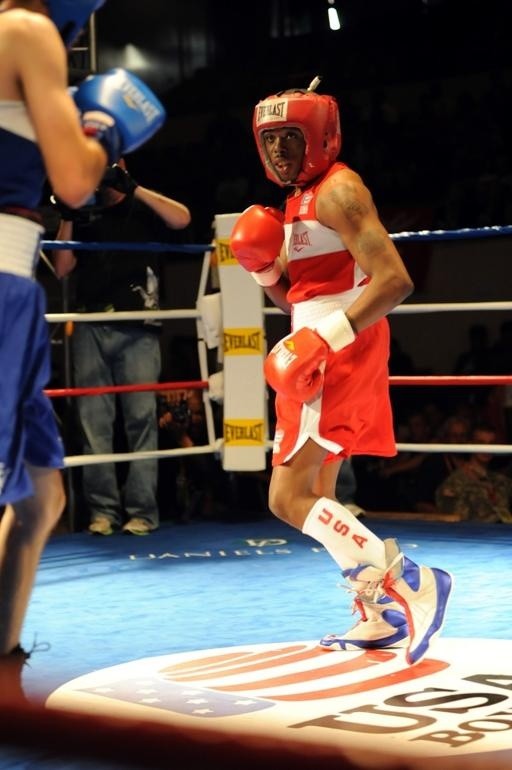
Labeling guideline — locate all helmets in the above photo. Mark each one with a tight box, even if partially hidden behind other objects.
[40,0,105,48]
[249,87,342,188]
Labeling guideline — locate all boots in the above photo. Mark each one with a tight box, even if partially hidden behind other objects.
[347,535,455,668]
[317,563,410,656]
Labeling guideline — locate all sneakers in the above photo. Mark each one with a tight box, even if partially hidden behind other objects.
[122,515,155,538]
[87,516,114,537]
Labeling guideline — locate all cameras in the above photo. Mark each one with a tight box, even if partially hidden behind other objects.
[160,394,193,429]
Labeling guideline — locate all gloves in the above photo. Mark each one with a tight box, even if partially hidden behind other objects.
[101,163,138,196]
[70,67,167,169]
[262,309,357,406]
[228,204,287,287]
[50,193,81,221]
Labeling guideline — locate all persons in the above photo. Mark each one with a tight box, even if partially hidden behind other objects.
[229,86,452,664]
[50,156,191,536]
[0,0,169,665]
